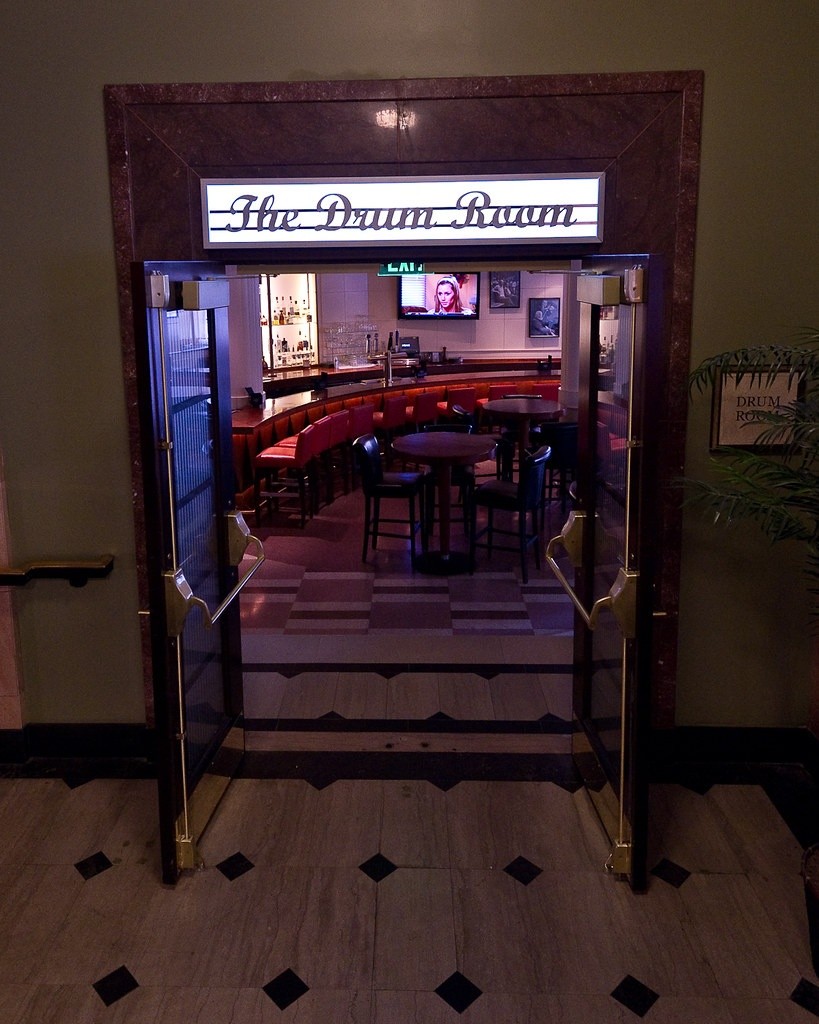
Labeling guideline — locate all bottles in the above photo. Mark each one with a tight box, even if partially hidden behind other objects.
[600,334,615,364]
[273,296,315,367]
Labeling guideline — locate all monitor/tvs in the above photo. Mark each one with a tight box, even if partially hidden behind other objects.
[399,336,420,354]
[397,271,482,320]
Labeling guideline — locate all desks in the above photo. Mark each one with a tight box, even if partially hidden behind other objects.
[230,369,560,507]
[482,398,563,482]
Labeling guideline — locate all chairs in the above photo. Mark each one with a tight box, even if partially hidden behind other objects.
[524,421,579,513]
[422,424,474,535]
[253,382,551,529]
[468,445,551,584]
[352,433,426,577]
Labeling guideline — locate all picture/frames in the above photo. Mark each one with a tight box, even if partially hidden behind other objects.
[489,271,521,309]
[528,297,561,338]
[709,364,807,456]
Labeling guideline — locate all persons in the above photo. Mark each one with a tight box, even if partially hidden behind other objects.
[492,272,519,305]
[427,276,471,314]
[532,300,556,335]
